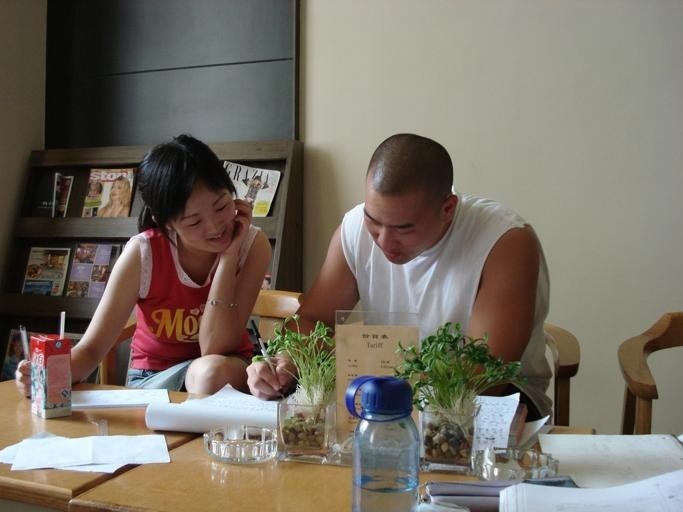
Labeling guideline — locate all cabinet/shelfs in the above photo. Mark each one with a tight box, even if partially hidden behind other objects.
[0,138,303,381]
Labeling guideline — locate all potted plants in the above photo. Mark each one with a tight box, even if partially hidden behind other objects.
[252,316,336,463]
[392,323,528,464]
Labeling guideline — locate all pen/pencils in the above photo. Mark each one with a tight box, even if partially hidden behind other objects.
[20,324,30,360]
[251,319,284,400]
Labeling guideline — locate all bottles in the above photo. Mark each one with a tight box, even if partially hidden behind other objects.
[344,375,421,511]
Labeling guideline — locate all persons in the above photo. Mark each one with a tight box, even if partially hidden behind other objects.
[244,131,558,425]
[12,133,272,401]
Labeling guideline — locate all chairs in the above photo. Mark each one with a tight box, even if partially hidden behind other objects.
[616,311,683,434]
[98,288,301,386]
[542,322,580,429]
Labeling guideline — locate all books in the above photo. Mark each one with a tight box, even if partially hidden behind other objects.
[64,241,127,299]
[220,157,281,218]
[67,387,172,412]
[497,473,682,512]
[20,246,71,298]
[79,167,138,218]
[412,385,532,457]
[420,476,572,510]
[143,379,286,438]
[203,297,239,313]
[0,325,41,383]
[58,329,104,385]
[28,172,73,219]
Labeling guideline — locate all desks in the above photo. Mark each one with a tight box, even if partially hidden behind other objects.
[68,428,615,512]
[0,376,210,512]
